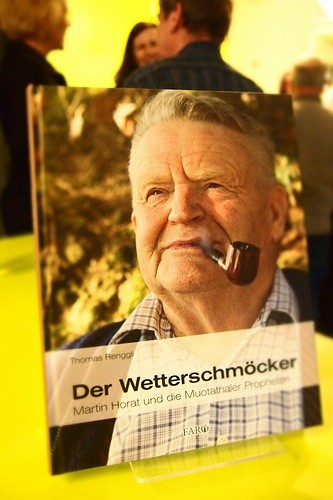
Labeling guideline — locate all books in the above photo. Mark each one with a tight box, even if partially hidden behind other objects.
[25,83,325,474]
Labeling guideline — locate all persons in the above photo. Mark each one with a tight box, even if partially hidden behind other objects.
[51,91,322,476]
[114,22,163,89]
[280,52,333,339]
[120,0,264,93]
[0,0,70,238]
